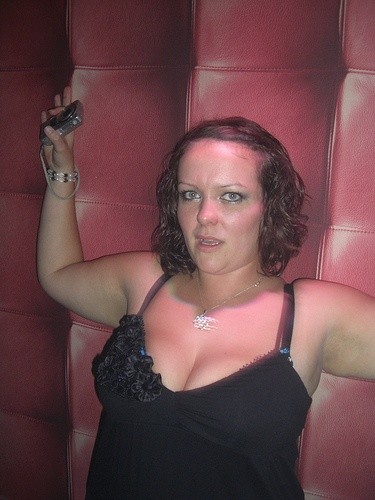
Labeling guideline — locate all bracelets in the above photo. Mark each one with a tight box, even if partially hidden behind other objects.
[45,170,77,185]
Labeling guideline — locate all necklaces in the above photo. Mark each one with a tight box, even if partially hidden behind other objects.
[193,269,261,331]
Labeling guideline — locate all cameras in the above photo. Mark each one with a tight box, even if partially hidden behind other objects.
[39,100,83,146]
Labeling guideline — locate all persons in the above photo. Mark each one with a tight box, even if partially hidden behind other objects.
[36,86,375,499]
